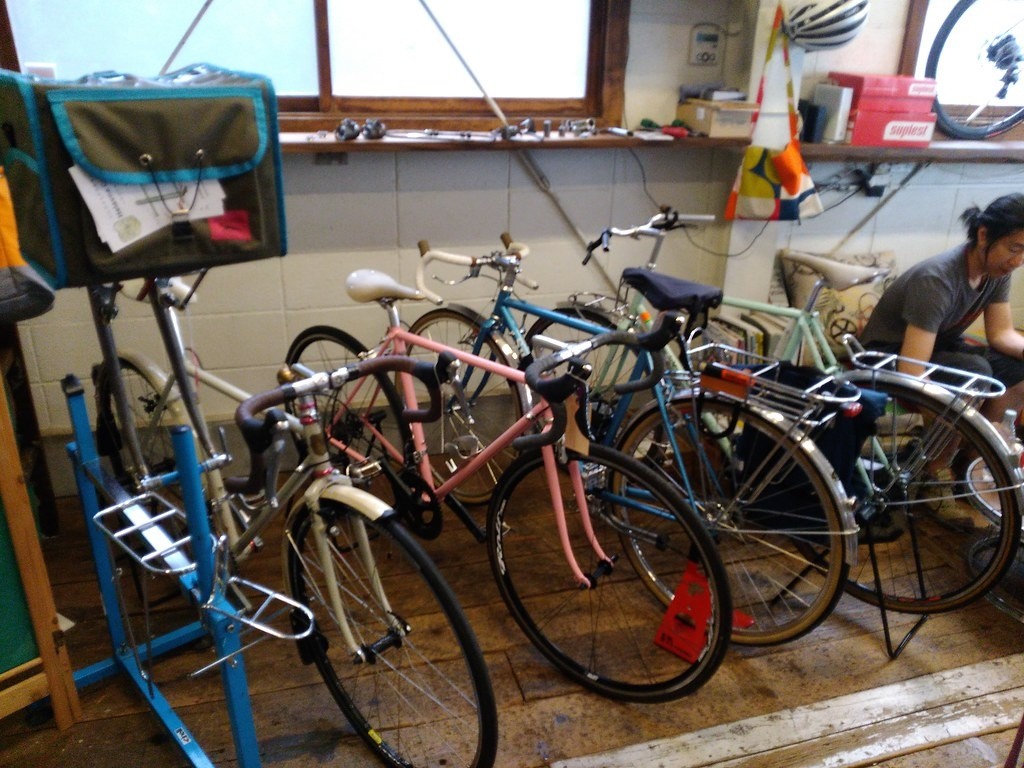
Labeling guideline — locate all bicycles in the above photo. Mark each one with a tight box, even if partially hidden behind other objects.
[91,274,500,768]
[524,203,1024,658]
[404,231,862,647]
[278,268,734,703]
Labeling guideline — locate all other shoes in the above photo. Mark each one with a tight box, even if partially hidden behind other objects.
[916,468,974,525]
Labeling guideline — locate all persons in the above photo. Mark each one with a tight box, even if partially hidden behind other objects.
[859,192,1024,515]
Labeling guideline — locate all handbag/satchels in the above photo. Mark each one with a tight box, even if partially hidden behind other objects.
[724,142,825,219]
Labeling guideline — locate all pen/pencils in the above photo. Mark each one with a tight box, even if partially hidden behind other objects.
[608,126,633,137]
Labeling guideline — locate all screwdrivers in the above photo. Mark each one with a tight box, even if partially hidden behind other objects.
[636,125,691,138]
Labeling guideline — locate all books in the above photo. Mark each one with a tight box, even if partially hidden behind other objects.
[702,309,805,366]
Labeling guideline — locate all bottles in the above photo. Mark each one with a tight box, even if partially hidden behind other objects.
[983,410,1017,482]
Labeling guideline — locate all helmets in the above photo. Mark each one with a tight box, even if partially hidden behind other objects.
[780,0,870,52]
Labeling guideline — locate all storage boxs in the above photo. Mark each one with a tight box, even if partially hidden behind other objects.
[842,109,938,148]
[676,99,761,138]
[829,72,939,113]
